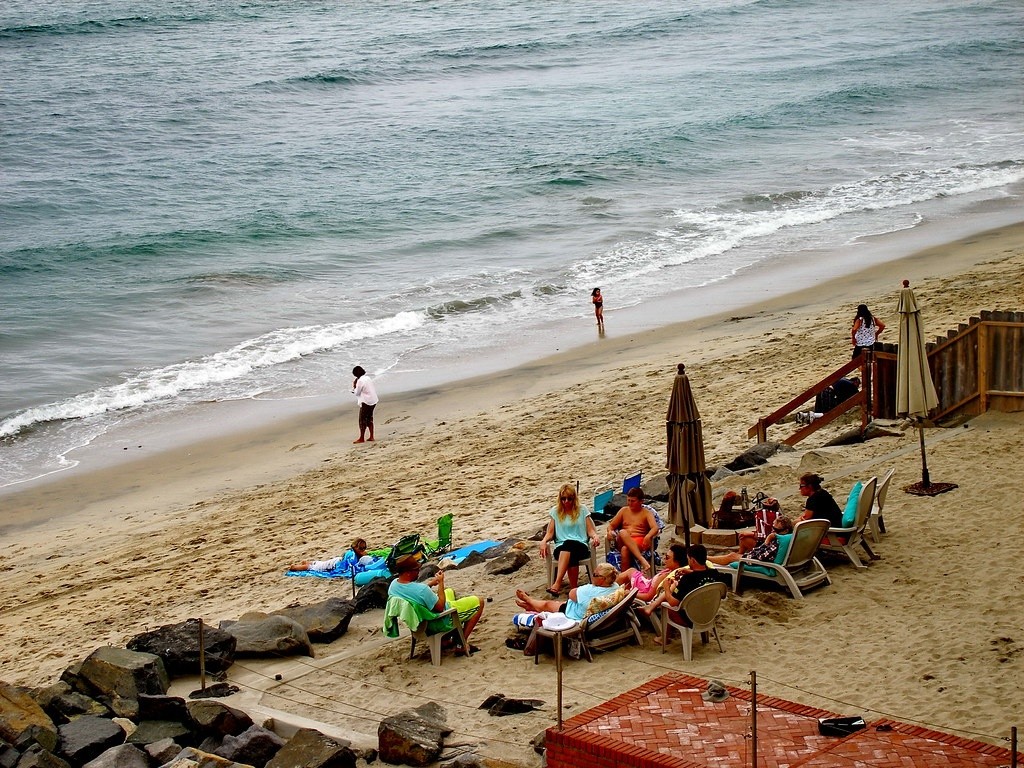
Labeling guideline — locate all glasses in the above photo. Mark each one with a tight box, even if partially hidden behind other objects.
[666,555,676,560]
[561,497,573,501]
[359,546,366,550]
[877,724,893,731]
[800,484,808,488]
[772,526,787,535]
[593,573,606,580]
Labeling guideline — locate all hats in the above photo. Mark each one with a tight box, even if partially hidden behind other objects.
[396,554,422,570]
[701,679,729,702]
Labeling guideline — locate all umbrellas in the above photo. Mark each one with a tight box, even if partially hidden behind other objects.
[895,279,939,486]
[664,361,715,544]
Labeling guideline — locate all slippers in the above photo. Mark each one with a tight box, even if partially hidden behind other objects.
[545,588,559,598]
[442,641,455,650]
[455,644,481,657]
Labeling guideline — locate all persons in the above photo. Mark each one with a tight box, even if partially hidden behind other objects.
[616,542,688,600]
[513,563,621,620]
[591,288,604,325]
[706,514,794,567]
[352,366,379,443]
[636,544,727,644]
[790,472,848,545]
[795,375,862,423]
[387,554,484,656]
[288,537,367,571]
[538,483,600,598]
[605,487,660,570]
[849,303,886,371]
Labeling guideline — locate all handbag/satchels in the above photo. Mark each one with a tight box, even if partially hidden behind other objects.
[711,510,756,529]
[751,492,785,516]
[818,716,867,735]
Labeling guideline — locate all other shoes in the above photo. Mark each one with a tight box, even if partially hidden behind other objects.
[505,638,527,650]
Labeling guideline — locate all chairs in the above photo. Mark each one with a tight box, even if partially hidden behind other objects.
[353,468,896,666]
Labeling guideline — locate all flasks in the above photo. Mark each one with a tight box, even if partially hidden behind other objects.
[741,486,749,510]
